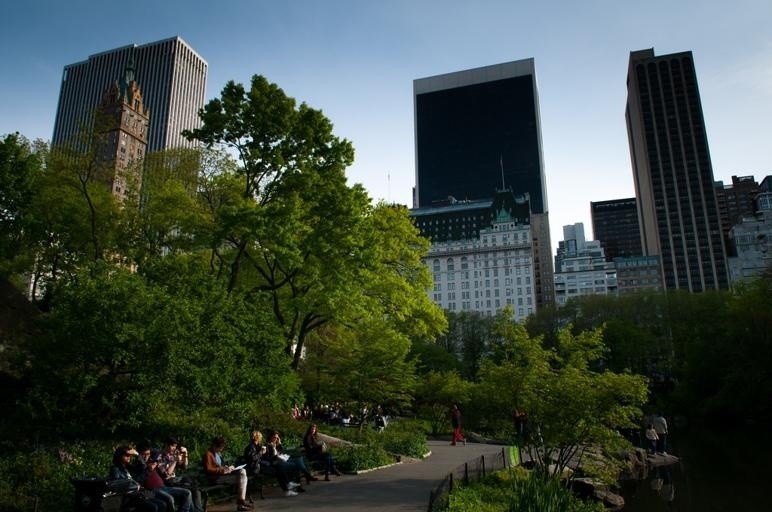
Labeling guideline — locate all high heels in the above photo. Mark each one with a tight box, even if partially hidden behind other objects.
[305,477,318,485]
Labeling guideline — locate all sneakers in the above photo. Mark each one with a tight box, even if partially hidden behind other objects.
[297,487,307,493]
[237,499,253,511]
[287,482,301,489]
[324,471,342,481]
[282,490,299,496]
[451,438,466,445]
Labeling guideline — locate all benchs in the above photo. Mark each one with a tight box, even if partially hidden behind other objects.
[195,476,266,512]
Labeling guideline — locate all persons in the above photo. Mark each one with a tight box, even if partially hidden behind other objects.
[645,422,659,455]
[292,400,390,433]
[515,406,529,440]
[450,404,466,445]
[109,422,344,512]
[654,408,668,456]
